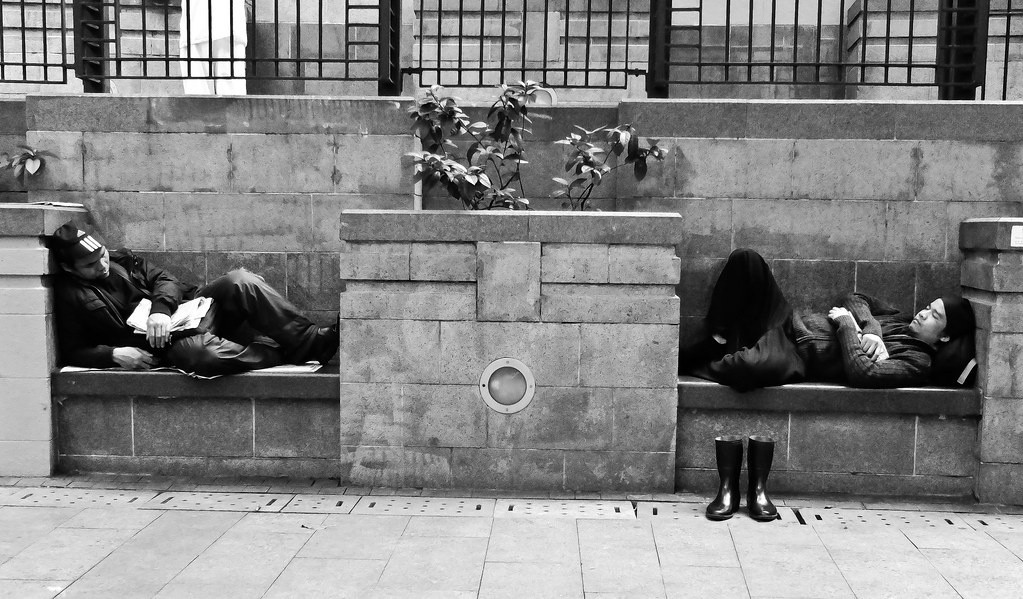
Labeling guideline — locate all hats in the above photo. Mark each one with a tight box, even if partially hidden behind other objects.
[50,218,106,263]
[938,295,975,342]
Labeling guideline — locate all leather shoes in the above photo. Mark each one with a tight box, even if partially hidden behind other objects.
[309,311,339,367]
[254,335,303,364]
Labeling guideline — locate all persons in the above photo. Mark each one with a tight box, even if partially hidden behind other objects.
[678,247,976,393]
[52,219,341,376]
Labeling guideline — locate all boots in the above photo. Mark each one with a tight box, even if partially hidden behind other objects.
[707,434,743,520]
[746,435,778,521]
